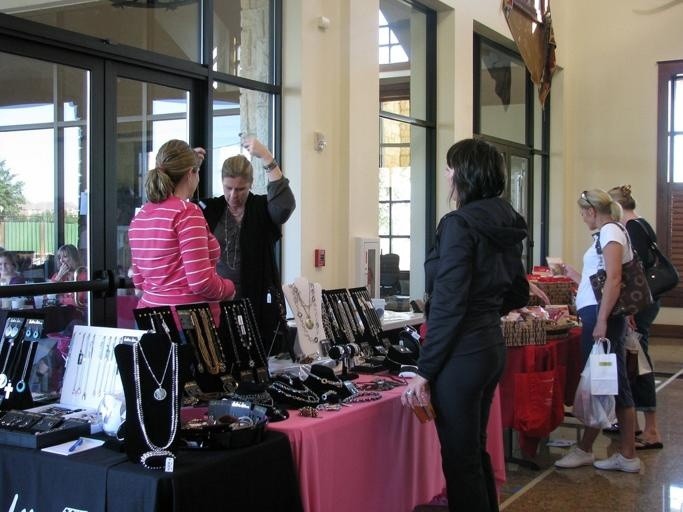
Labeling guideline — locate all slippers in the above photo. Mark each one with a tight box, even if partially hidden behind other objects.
[602,423,642,436]
[634,437,663,450]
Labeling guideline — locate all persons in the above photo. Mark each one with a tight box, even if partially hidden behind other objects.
[127,140,237,332]
[47,244,88,306]
[554,188,642,474]
[198,131,295,335]
[607,184,664,452]
[0,250,37,308]
[401,140,533,512]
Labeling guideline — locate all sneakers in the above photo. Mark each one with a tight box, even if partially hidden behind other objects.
[554,447,595,468]
[592,453,641,473]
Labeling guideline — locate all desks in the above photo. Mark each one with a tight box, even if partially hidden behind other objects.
[498,300,583,464]
[0,405,294,511]
[364,304,427,342]
[180,348,506,512]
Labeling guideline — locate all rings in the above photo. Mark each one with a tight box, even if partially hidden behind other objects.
[405,390,414,396]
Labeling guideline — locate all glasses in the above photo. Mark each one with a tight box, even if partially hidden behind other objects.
[580,191,597,213]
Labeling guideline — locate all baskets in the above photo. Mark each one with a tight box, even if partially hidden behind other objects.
[545,319,572,340]
[498,320,547,341]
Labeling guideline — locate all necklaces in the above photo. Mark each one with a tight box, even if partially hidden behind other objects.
[131,304,298,472]
[2,316,133,458]
[225,203,245,271]
[229,205,244,217]
[297,270,401,419]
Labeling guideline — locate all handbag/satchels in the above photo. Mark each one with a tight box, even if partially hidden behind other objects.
[625,348,639,378]
[513,343,564,438]
[589,337,619,396]
[589,222,654,320]
[632,219,679,298]
[626,324,652,377]
